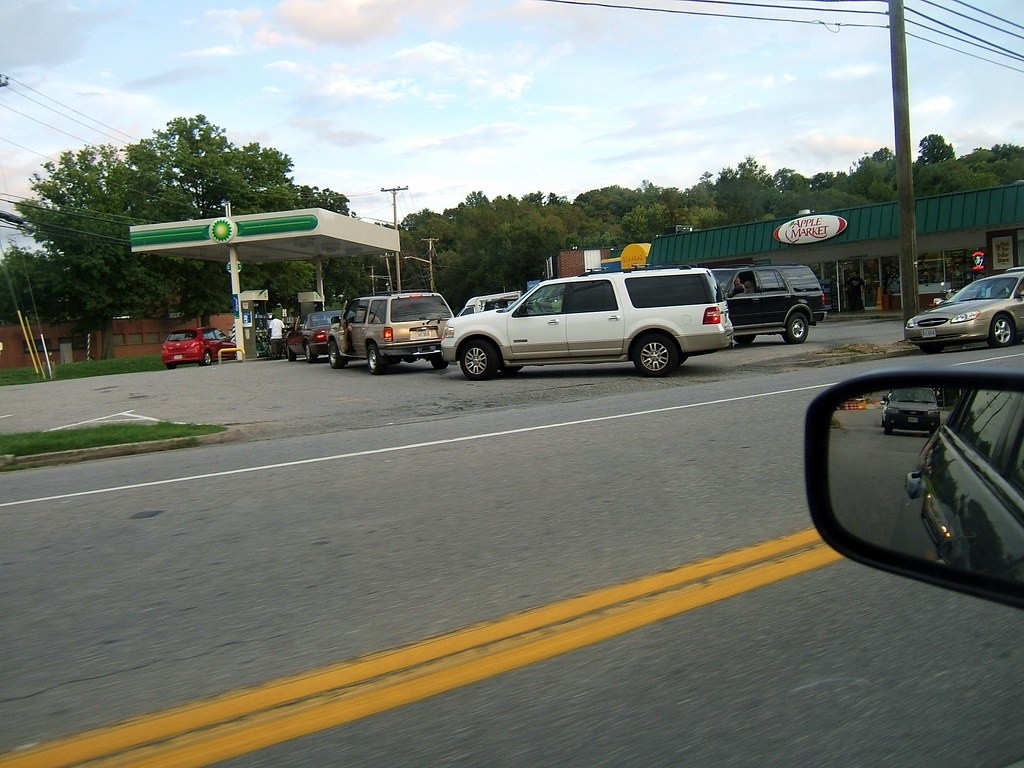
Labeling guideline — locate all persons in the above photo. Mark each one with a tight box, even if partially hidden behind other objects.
[733,276,746,294]
[537,287,565,312]
[845,272,866,314]
[268,314,286,359]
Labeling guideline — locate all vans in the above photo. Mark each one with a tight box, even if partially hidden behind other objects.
[456,290,525,317]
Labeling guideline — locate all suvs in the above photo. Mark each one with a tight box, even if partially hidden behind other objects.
[880,386,941,436]
[326,288,455,375]
[712,264,828,345]
[439,263,737,381]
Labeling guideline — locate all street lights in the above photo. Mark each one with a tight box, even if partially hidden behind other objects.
[404,256,433,291]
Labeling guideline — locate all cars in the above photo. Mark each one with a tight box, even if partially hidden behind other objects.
[284,310,359,364]
[904,266,1024,355]
[162,326,237,370]
